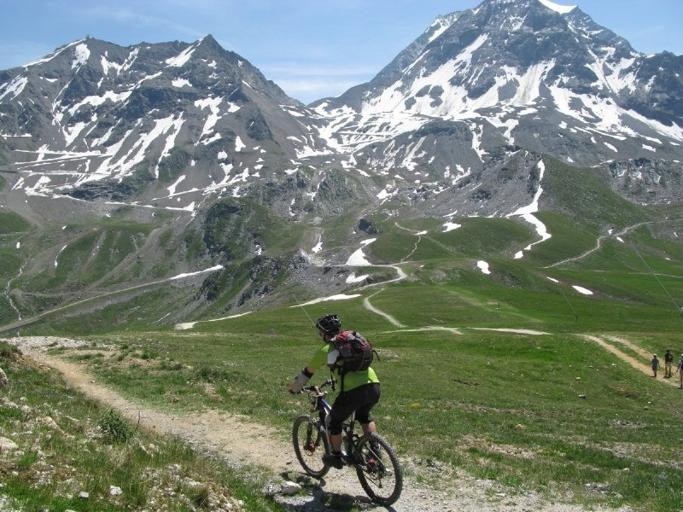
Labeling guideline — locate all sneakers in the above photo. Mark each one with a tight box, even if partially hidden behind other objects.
[365,444,382,460]
[323,452,343,469]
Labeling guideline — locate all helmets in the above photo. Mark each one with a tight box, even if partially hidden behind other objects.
[316,314,342,335]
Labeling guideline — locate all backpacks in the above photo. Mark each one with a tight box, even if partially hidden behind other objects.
[328,330,373,370]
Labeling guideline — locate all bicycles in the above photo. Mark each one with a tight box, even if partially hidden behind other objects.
[290,378,403,507]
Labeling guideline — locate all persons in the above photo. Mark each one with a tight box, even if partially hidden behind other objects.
[663,350,673,377]
[650,354,660,377]
[676,354,683,389]
[287,314,382,473]
